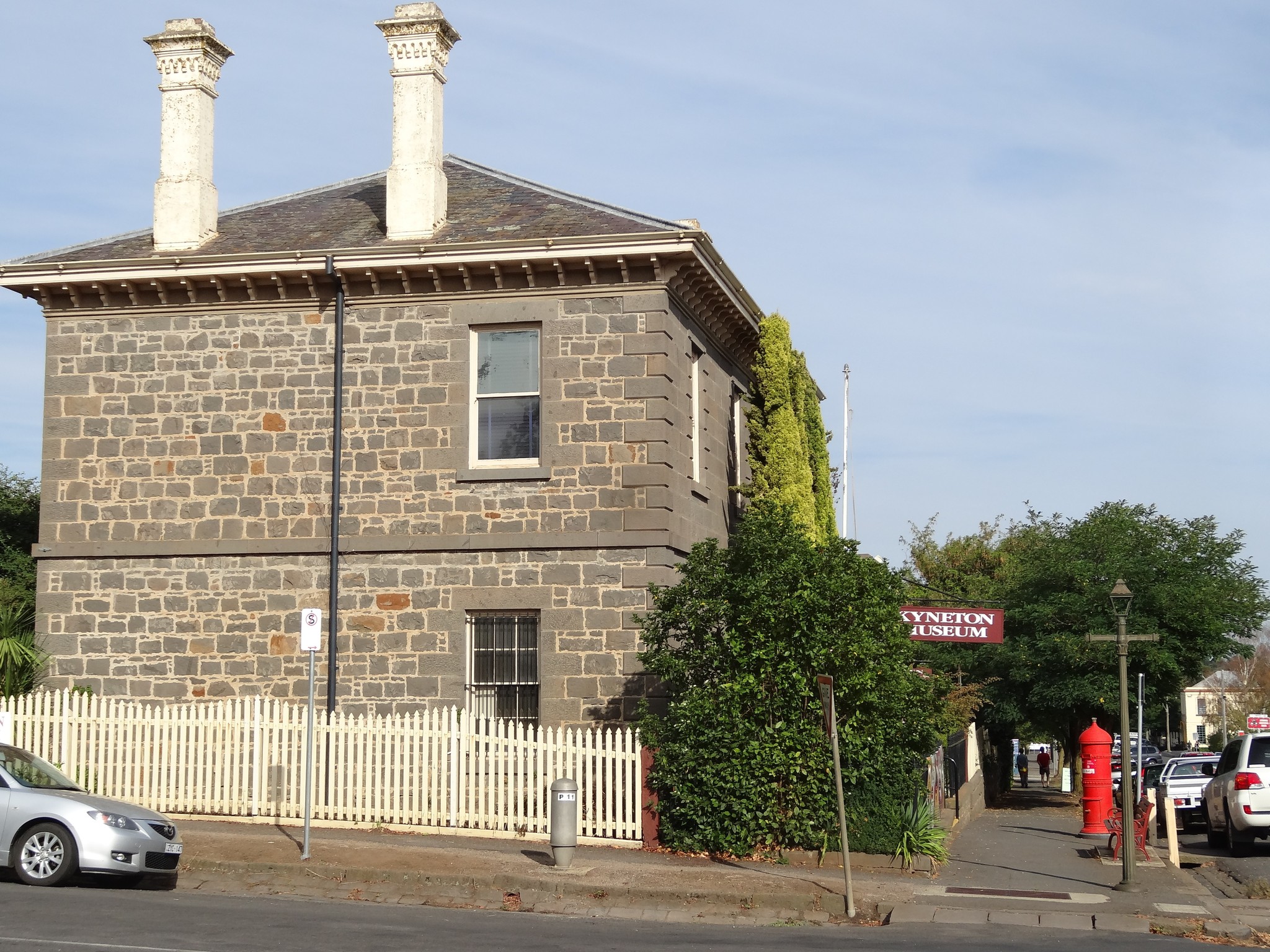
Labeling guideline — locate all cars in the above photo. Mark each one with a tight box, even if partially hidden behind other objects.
[0,742,184,887]
[1111,732,1223,836]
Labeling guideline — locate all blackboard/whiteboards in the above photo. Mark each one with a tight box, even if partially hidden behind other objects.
[1061,768,1071,793]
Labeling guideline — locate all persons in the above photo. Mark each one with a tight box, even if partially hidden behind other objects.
[1187,741,1192,750]
[1037,747,1051,789]
[1194,741,1200,749]
[1016,748,1029,788]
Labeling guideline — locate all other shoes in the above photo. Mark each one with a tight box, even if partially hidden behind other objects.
[1021,786,1025,788]
[1047,786,1051,788]
[1025,786,1030,788]
[1043,786,1046,788]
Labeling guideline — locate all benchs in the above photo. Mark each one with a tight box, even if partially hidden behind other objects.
[1104,795,1155,862]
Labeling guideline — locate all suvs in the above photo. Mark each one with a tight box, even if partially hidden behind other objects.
[1200,732,1270,858]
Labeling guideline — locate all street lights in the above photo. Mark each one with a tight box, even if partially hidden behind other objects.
[1084,577,1160,886]
